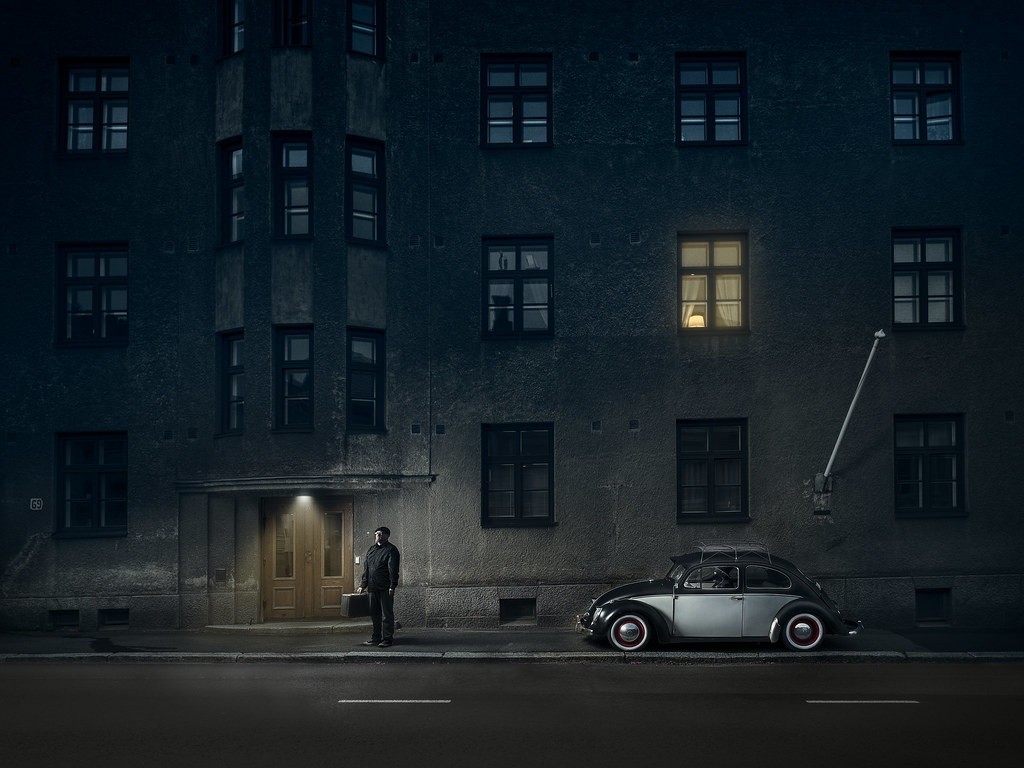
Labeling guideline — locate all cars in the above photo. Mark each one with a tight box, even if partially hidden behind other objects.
[576,538,863,652]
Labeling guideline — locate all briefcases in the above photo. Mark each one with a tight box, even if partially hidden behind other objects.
[341,590,370,618]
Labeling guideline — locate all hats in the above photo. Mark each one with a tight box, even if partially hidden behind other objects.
[375,527,389,531]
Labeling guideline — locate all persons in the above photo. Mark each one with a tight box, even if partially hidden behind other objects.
[354,526,400,647]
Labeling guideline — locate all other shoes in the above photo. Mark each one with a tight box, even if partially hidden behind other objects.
[364,637,393,647]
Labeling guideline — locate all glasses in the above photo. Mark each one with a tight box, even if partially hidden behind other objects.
[374,532,389,534]
